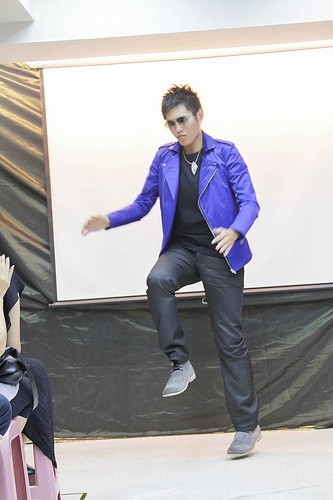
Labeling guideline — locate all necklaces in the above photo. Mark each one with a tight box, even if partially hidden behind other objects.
[181,147,202,176]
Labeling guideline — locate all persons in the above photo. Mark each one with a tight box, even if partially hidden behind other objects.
[0,255,36,476]
[81,84,263,457]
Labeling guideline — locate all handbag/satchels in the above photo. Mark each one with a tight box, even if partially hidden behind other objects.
[0,347,39,410]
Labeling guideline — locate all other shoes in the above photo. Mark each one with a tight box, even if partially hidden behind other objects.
[162,360,196,397]
[227,424,262,457]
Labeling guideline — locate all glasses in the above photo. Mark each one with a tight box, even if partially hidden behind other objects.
[164,110,197,128]
